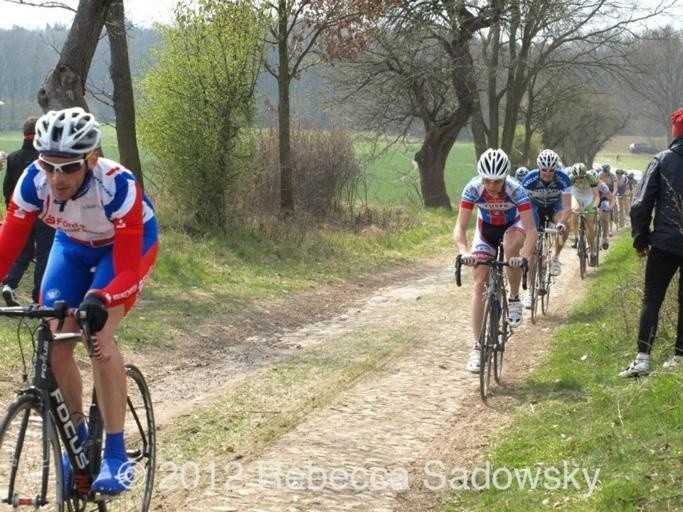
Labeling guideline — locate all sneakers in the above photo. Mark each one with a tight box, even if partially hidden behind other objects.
[662,354,682,369]
[91,455,136,497]
[618,359,651,378]
[571,236,578,249]
[550,256,562,276]
[507,301,524,328]
[602,239,609,250]
[466,347,483,373]
[60,450,74,501]
[589,255,596,266]
[524,294,534,310]
[2,284,22,307]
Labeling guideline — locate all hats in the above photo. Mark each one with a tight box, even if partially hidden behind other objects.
[563,162,635,179]
[476,147,513,181]
[515,167,529,180]
[32,106,103,160]
[22,116,39,136]
[536,148,558,170]
[670,108,683,139]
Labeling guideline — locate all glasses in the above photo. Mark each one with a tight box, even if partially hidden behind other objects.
[37,149,98,175]
[484,179,503,185]
[542,169,556,174]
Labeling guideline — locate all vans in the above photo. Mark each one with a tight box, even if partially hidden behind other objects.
[627,142,660,157]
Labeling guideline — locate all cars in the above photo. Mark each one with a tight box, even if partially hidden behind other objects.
[625,169,643,188]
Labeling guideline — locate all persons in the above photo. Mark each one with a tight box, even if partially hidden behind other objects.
[516,147,638,311]
[0,108,159,499]
[454,147,540,373]
[3,116,56,306]
[0,152,7,169]
[619,109,683,378]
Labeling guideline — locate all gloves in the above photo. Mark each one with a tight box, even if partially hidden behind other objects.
[459,251,479,268]
[555,221,569,234]
[76,293,110,337]
[507,256,527,270]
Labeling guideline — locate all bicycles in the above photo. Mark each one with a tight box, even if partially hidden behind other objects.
[453,193,635,400]
[0,300,154,511]
